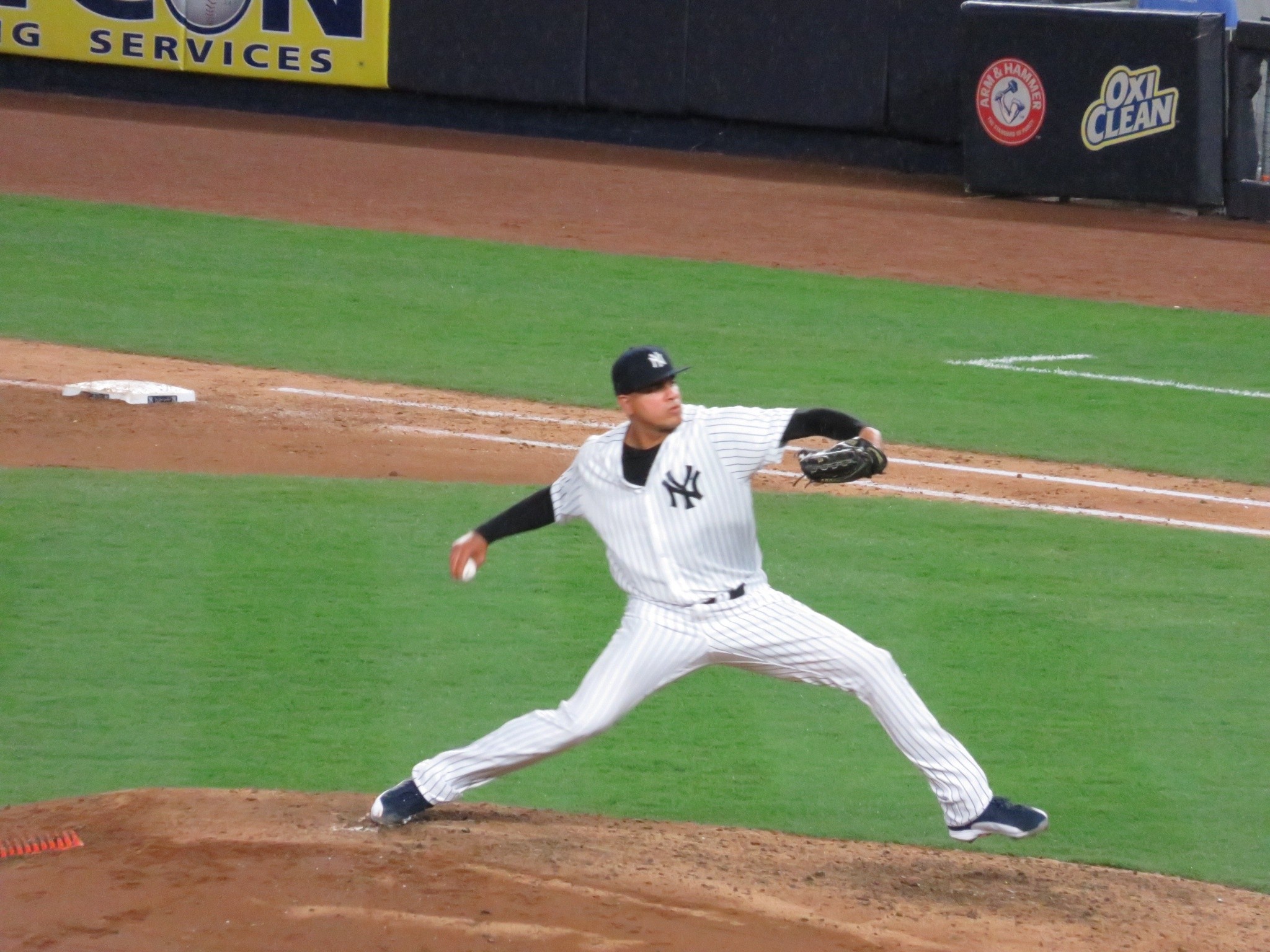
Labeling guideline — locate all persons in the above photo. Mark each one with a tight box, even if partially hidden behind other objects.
[369,344,1048,840]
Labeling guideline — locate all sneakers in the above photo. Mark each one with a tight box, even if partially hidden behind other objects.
[947,796,1050,844]
[369,775,433,826]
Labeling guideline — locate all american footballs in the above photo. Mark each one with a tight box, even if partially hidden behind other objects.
[463,556,477,583]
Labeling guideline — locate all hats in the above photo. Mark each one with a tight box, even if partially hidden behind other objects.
[610,346,693,397]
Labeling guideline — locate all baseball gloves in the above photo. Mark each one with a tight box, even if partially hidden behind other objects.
[795,436,887,483]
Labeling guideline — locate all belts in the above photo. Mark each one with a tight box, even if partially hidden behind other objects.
[700,583,745,605]
